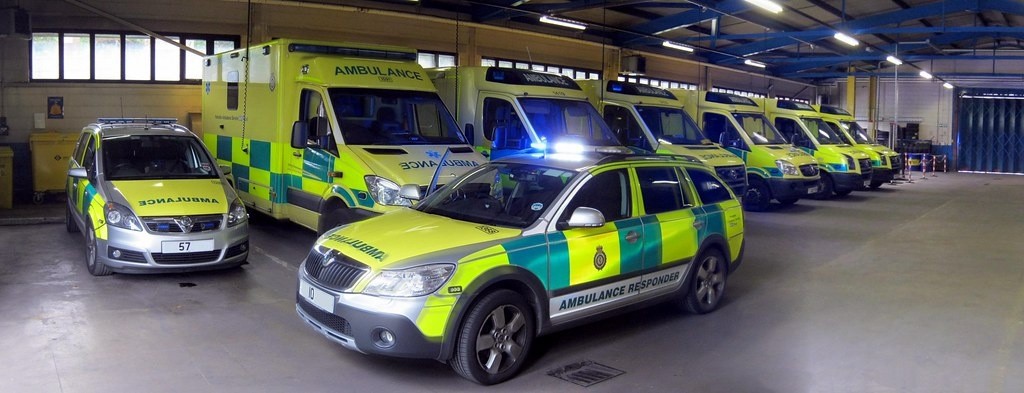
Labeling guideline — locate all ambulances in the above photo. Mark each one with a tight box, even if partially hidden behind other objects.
[573,79,747,197]
[665,88,821,212]
[749,98,873,200]
[423,65,622,189]
[201,38,489,240]
[65,118,250,276]
[811,105,903,187]
[295,143,745,386]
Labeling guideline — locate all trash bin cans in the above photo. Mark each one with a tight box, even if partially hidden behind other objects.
[0,146,15,209]
[29,130,81,205]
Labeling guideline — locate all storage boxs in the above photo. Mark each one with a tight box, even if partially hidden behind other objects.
[0,146,14,209]
[28,132,82,191]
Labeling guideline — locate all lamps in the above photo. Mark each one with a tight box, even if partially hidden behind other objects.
[833,31,860,46]
[662,40,695,53]
[746,0,783,14]
[886,54,903,66]
[539,15,589,30]
[920,70,933,79]
[942,82,953,89]
[744,59,766,68]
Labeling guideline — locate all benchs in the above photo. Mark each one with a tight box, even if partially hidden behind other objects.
[641,188,678,214]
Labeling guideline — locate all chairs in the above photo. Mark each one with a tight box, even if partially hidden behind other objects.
[563,174,620,223]
[531,111,551,143]
[509,173,563,220]
[492,106,518,149]
[371,105,403,143]
[162,146,189,174]
